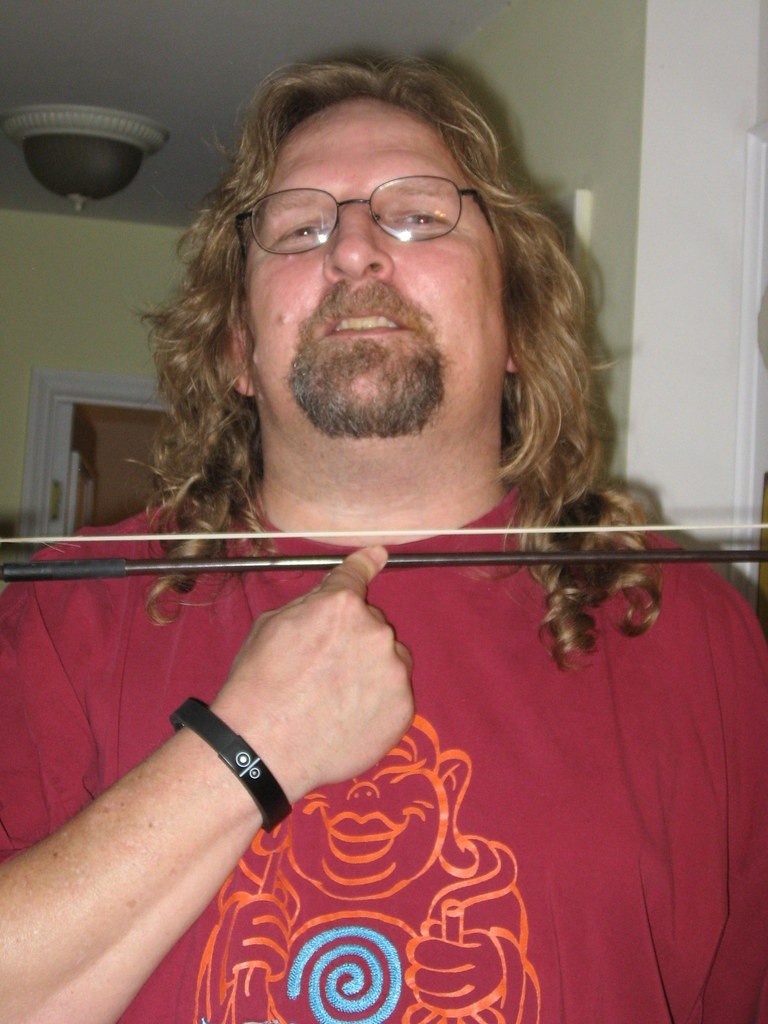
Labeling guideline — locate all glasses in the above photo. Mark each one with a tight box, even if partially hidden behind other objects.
[235,175,495,266]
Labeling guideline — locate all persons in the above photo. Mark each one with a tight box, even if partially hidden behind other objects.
[0,44,768,1023]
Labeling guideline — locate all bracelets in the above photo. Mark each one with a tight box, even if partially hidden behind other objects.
[171,697,292,832]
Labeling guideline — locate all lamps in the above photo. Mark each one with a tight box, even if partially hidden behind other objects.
[0,102,170,209]
